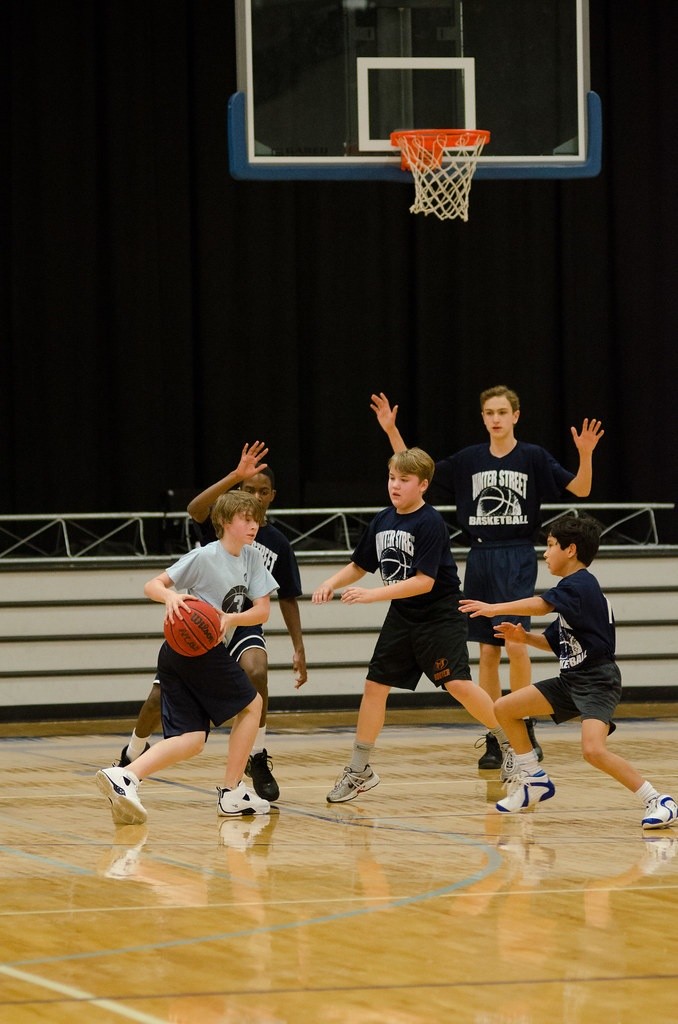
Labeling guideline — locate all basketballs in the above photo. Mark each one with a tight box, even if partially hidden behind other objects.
[164,599,221,658]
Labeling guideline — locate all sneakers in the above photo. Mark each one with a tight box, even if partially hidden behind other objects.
[474,732,503,769]
[499,744,521,783]
[327,764,380,803]
[641,794,678,828]
[216,780,270,816]
[244,748,280,801]
[113,741,151,782]
[495,770,556,813]
[94,763,147,824]
[524,717,544,762]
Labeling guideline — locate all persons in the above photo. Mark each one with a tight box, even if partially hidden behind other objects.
[371,387,604,768]
[458,517,678,828]
[95,491,279,824]
[312,447,520,803]
[113,441,308,802]
